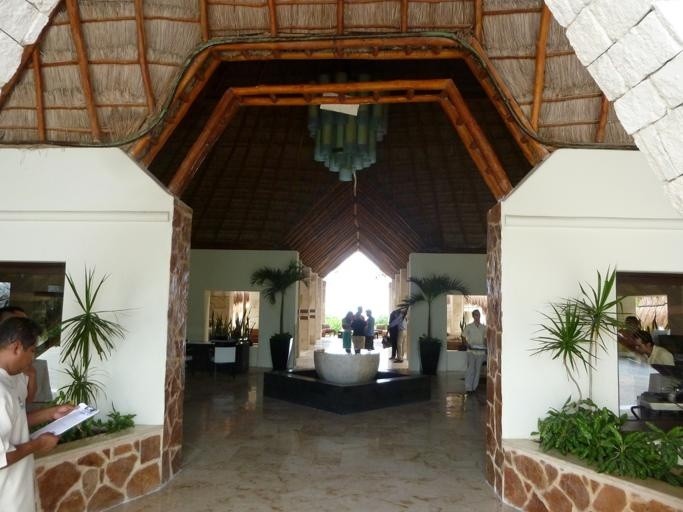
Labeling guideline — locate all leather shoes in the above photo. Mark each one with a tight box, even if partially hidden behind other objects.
[389,357,403,363]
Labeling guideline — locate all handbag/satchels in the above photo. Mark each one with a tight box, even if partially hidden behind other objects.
[382,336,392,349]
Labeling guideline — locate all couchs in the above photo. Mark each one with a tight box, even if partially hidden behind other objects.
[322,324,336,337]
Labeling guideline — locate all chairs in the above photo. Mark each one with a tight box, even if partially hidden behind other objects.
[209,346,237,379]
[374,325,385,334]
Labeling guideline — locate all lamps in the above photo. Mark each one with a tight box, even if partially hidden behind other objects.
[309,73,385,184]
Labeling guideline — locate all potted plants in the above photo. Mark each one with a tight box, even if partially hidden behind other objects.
[211,310,232,340]
[395,272,471,376]
[248,255,311,371]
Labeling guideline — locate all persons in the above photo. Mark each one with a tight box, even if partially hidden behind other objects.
[632,329,676,366]
[341,311,354,354]
[460,309,488,396]
[386,309,407,363]
[0,316,81,512]
[350,312,367,353]
[0,305,37,405]
[617,315,641,356]
[386,310,399,360]
[364,310,374,351]
[353,306,364,321]
